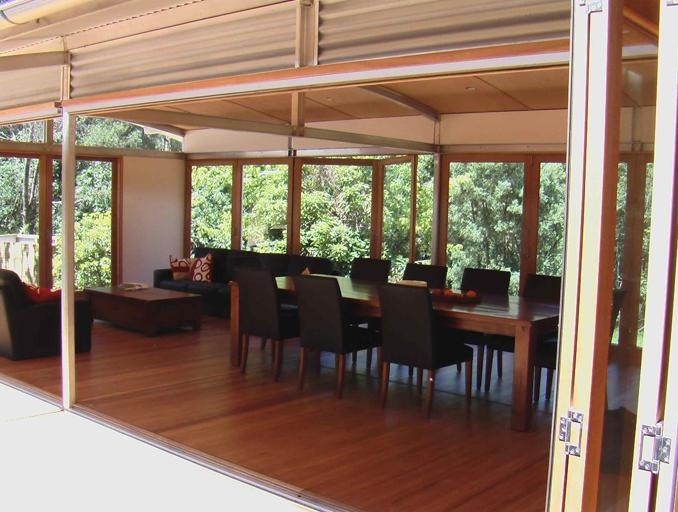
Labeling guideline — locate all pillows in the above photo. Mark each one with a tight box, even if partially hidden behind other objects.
[169,254,193,281]
[190,253,213,283]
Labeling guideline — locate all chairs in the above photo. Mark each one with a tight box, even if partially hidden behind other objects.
[611,288,628,340]
[0,269,92,361]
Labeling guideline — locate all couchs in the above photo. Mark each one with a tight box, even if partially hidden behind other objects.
[153,247,331,336]
